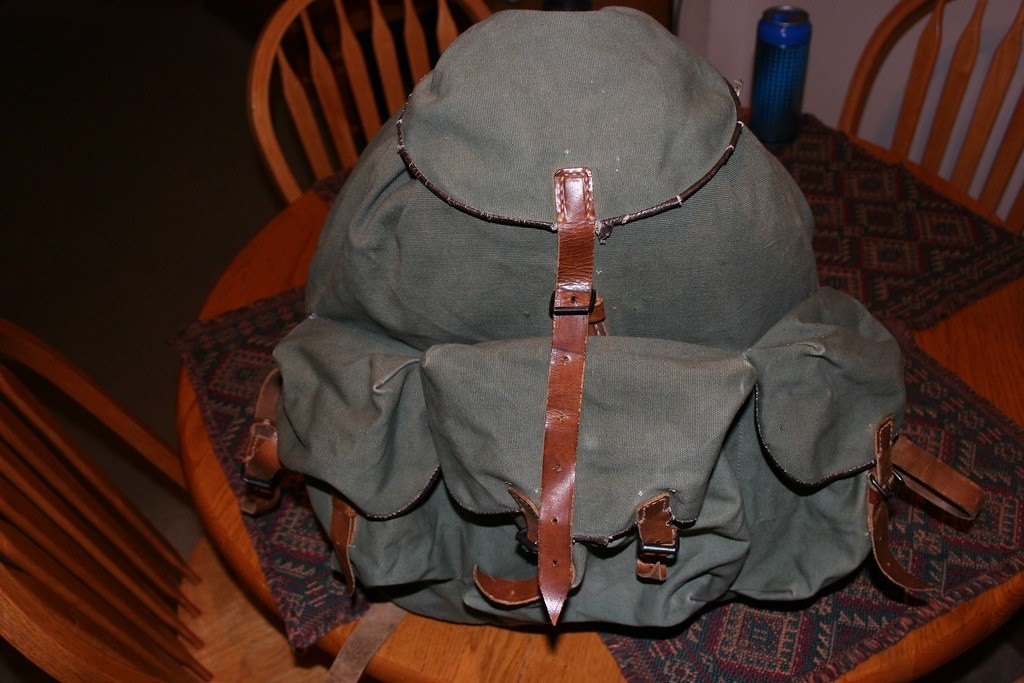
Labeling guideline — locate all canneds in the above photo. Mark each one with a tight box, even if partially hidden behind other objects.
[750,5,812,145]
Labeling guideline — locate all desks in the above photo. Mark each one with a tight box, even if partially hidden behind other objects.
[177,108,1024,683]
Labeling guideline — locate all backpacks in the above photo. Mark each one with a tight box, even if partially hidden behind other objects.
[237,6,982,631]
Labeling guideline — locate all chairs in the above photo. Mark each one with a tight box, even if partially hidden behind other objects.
[251,0,495,211]
[0,314,357,683]
[836,0,1024,237]
